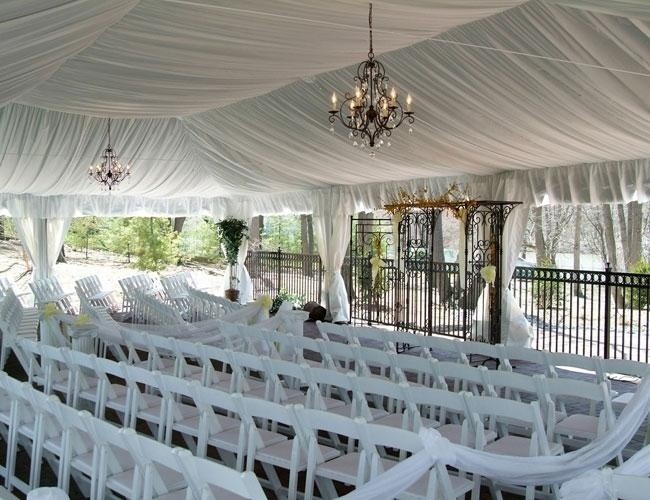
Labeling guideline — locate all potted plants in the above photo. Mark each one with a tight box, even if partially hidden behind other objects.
[214,215,251,301]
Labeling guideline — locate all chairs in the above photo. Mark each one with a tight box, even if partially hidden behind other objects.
[308,321,650,500]
[0,273,354,500]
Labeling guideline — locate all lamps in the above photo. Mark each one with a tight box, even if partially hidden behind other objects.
[88,117,131,189]
[325,1,420,159]
